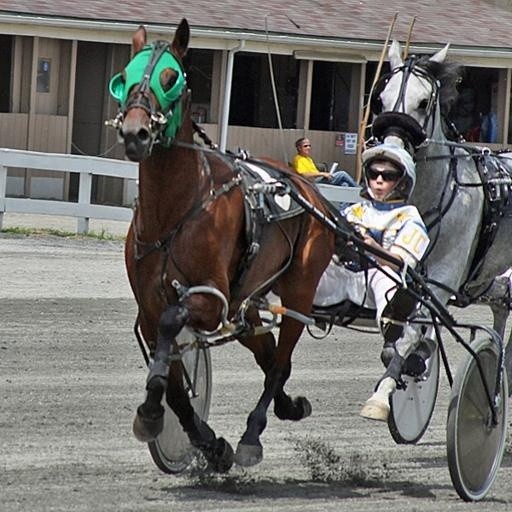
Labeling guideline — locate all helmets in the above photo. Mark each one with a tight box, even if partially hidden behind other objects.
[360,143,418,203]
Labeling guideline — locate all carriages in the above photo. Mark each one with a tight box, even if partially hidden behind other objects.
[107,19,509,501]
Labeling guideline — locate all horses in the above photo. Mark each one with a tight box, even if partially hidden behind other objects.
[360,39,512,422]
[117,18,336,473]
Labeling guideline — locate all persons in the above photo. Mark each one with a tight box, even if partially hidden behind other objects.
[311,146,431,342]
[293,136,358,187]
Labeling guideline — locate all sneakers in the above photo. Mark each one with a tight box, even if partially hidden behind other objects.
[379,285,419,343]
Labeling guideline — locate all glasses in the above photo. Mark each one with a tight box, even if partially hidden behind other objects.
[364,167,400,183]
